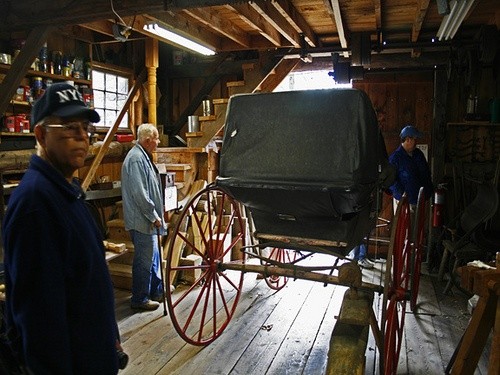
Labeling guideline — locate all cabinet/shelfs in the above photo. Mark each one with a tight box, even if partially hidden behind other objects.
[0,63,91,137]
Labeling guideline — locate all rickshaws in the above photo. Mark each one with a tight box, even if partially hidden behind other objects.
[165,89,432,375]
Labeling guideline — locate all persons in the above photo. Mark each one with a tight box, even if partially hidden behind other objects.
[352,127,436,269]
[121,124,174,311]
[4,84,125,375]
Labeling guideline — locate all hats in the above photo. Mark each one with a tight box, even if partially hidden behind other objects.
[399,126,427,138]
[32,82,101,125]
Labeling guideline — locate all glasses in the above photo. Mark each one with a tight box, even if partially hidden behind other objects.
[45,121,96,134]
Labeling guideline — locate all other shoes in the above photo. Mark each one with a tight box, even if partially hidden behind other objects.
[131,297,166,310]
[357,260,374,269]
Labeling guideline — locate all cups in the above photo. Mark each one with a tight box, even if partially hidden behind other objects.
[188,116,199,133]
[202,100,214,116]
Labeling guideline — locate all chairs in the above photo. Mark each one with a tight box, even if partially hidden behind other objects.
[215,89,388,216]
